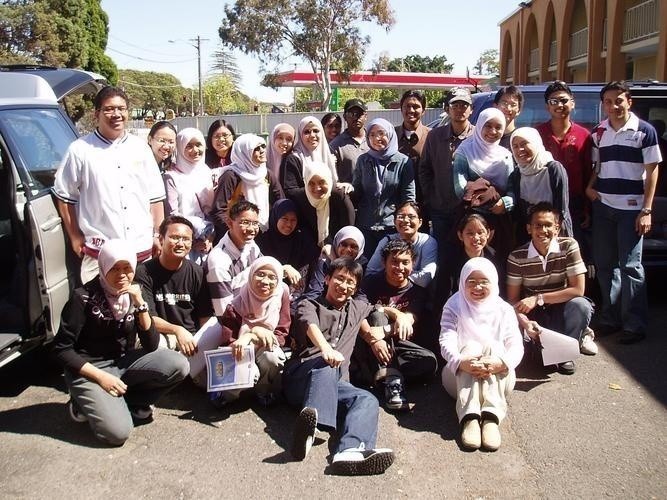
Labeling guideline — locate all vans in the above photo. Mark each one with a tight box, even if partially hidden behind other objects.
[420,77,667,275]
[0,63,110,372]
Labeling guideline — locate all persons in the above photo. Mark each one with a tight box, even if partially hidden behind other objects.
[281,256,397,477]
[437,257,523,450]
[58,238,194,448]
[50,77,662,411]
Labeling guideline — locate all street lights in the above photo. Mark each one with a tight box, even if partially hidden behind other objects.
[167,35,203,117]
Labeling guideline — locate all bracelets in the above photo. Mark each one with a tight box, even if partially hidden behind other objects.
[133,301,149,314]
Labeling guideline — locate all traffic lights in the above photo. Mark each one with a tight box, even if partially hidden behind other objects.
[182,95,187,102]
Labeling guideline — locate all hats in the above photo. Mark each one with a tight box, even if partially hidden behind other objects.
[344,99,368,113]
[446,87,473,105]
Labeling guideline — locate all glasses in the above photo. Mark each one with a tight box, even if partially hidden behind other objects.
[212,134,232,140]
[498,102,518,108]
[344,112,366,117]
[547,98,572,105]
[466,279,489,287]
[95,105,127,114]
